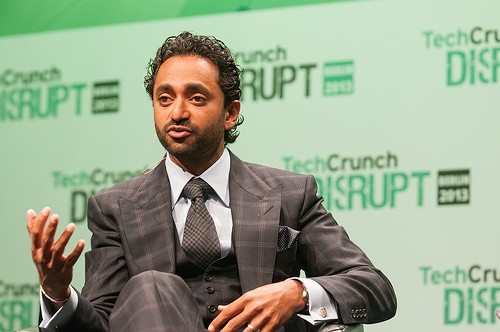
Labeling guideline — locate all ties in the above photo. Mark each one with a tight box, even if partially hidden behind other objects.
[181,178,222,263]
